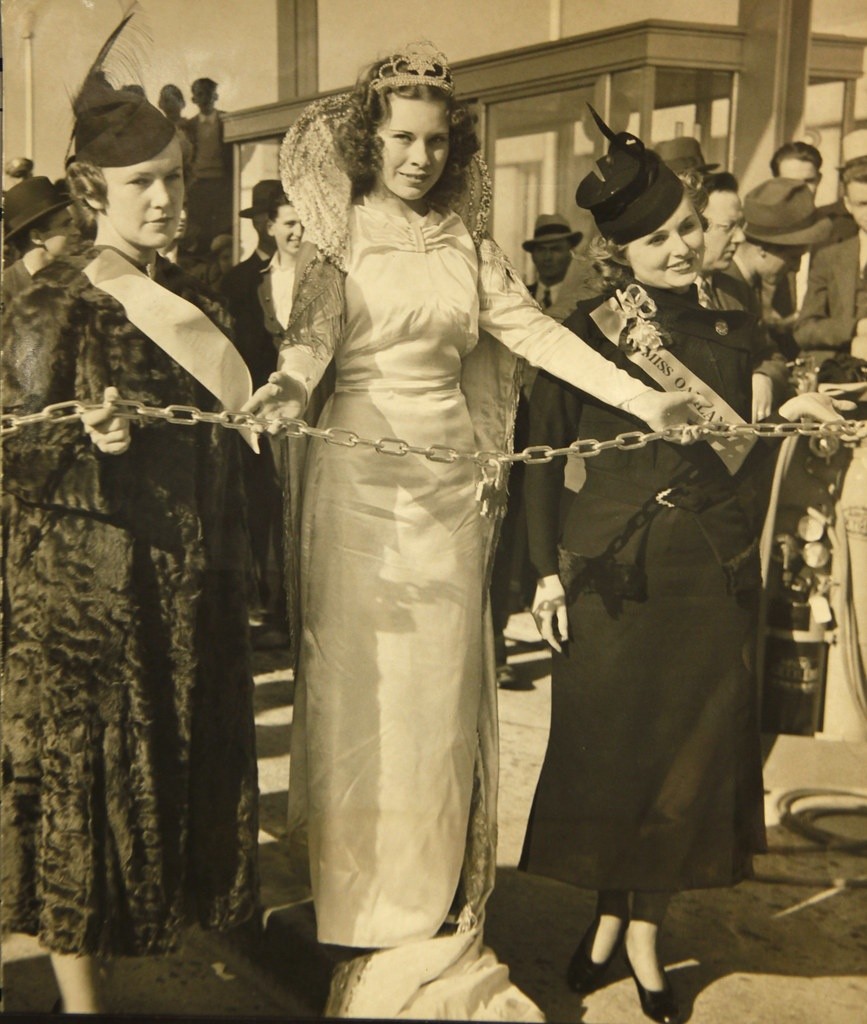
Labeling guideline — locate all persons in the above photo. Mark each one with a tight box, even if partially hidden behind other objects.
[516,150,857,1024]
[1,157,82,300]
[667,136,866,722]
[491,215,584,689]
[0,67,260,1013]
[160,76,322,634]
[237,40,712,1021]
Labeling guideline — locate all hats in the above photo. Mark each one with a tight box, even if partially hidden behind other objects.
[522,215,582,252]
[74,87,176,167]
[654,136,720,174]
[237,178,288,218]
[575,101,684,244]
[4,177,77,242]
[741,177,833,245]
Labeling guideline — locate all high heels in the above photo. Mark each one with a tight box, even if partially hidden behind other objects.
[568,918,623,990]
[623,938,677,1024]
